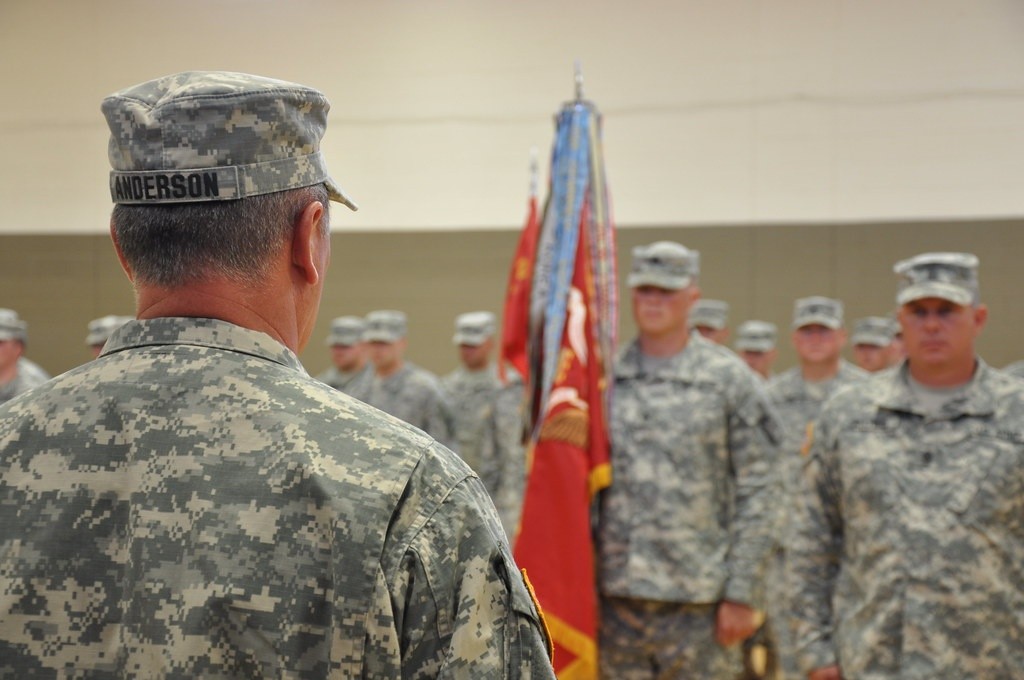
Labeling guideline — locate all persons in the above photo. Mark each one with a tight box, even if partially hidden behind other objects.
[783,249,1024,680]
[689,294,1024,578]
[0,308,53,411]
[589,239,788,680]
[0,71,556,680]
[86,310,529,554]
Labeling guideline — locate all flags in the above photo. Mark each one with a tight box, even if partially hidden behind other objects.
[499,100,618,680]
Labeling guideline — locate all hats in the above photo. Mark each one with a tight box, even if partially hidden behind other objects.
[0,309,26,342]
[326,316,363,345]
[690,300,729,329]
[629,241,701,290]
[735,322,779,349]
[894,252,981,307]
[451,312,496,347]
[853,317,893,346]
[85,316,136,345]
[362,310,407,343]
[100,70,359,212]
[792,297,843,331]
